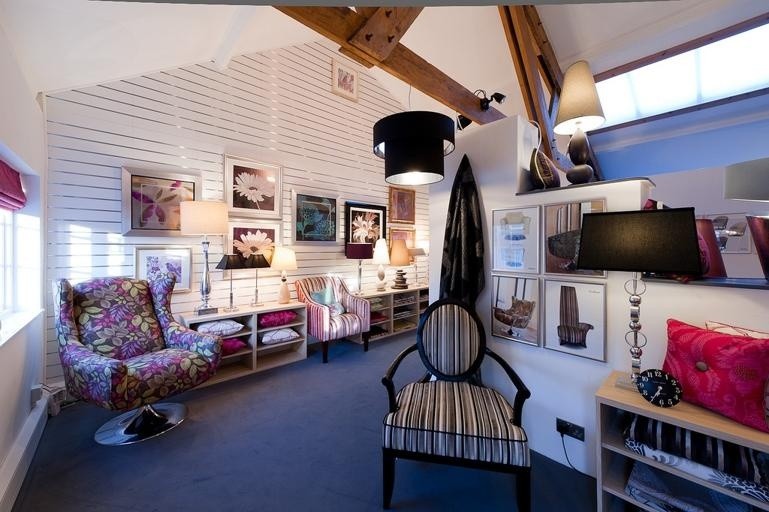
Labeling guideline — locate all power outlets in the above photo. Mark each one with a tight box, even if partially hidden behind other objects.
[555,417,585,441]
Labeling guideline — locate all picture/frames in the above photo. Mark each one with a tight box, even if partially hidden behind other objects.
[120,165,202,238]
[345,201,386,257]
[290,187,340,246]
[331,57,359,103]
[390,226,417,262]
[224,153,284,221]
[542,197,609,279]
[490,204,541,275]
[388,186,416,224]
[542,278,607,363]
[134,246,192,295]
[490,273,541,347]
[227,223,280,269]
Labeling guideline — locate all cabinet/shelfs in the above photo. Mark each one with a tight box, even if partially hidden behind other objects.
[596,370,769,512]
[179,301,307,392]
[353,283,429,345]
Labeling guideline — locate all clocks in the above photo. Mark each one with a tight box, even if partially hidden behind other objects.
[636,368,683,409]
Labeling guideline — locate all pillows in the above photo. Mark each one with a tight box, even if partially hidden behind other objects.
[663,319,769,434]
[197,319,244,336]
[260,311,297,328]
[704,320,769,340]
[259,328,300,345]
[222,339,247,355]
[310,286,346,316]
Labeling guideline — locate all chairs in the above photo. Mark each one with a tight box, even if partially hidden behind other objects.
[381,298,532,512]
[53,269,223,446]
[296,274,371,364]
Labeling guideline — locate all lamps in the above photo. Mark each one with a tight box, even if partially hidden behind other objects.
[408,249,427,263]
[373,86,456,186]
[271,247,297,304]
[215,254,243,312]
[552,60,608,185]
[347,242,372,295]
[180,200,230,316]
[391,239,410,289]
[479,93,506,111]
[372,238,389,292]
[576,207,706,394]
[245,253,271,307]
